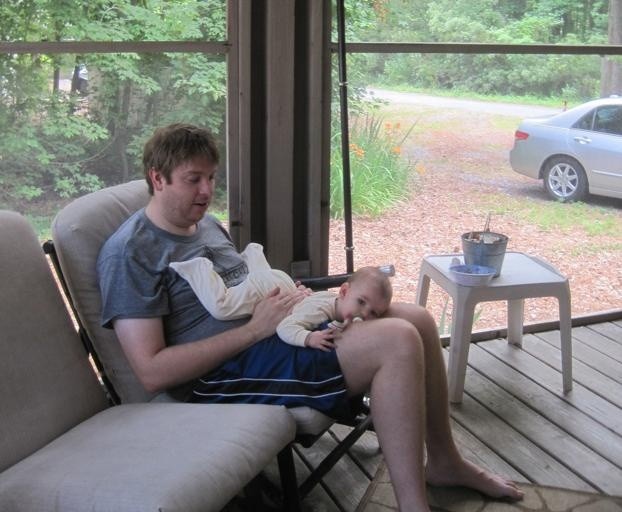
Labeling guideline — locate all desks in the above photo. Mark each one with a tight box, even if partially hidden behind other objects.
[411,248,575,407]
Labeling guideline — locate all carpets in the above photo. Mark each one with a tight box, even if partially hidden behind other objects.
[351,447,621,512]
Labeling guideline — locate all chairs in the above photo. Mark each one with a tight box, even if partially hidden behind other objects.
[36,173,398,512]
[0,207,301,512]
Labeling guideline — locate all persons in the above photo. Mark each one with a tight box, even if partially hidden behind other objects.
[95,122,524,512]
[169,243,393,354]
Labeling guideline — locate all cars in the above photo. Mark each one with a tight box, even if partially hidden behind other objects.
[71,62,97,95]
[509,97,622,204]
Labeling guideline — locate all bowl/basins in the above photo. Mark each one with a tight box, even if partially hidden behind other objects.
[450,263,499,287]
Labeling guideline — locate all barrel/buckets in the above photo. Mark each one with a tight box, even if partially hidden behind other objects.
[460,210,509,279]
